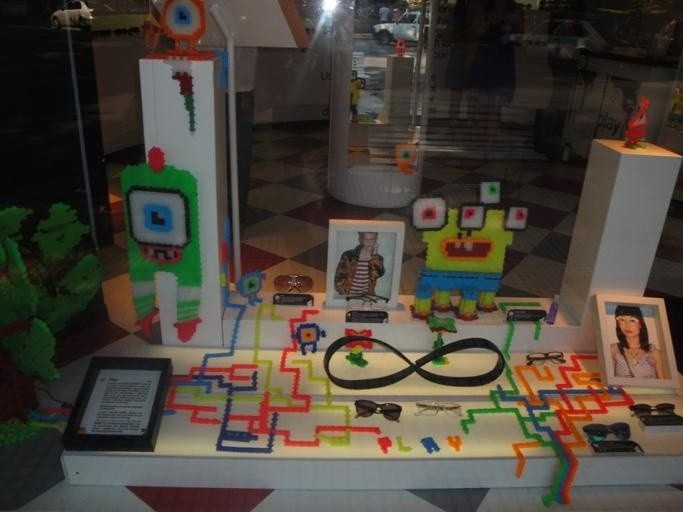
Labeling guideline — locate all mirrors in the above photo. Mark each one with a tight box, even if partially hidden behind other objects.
[347,1,431,178]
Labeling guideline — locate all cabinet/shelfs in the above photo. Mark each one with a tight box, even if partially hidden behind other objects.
[560,53,676,162]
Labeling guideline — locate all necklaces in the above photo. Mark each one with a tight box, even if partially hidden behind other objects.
[628,345,641,365]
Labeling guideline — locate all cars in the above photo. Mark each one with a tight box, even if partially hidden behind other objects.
[371,11,429,46]
[510,18,610,60]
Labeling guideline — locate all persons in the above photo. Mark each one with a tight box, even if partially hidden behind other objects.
[334,233,384,296]
[610,306,665,379]
[441,0,658,167]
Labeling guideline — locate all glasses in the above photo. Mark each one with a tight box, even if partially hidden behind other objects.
[525,351,566,366]
[414,399,462,417]
[273,273,313,293]
[344,291,388,311]
[582,422,630,442]
[628,402,676,417]
[353,399,401,423]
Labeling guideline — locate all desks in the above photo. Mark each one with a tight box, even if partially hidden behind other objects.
[61,349,683,490]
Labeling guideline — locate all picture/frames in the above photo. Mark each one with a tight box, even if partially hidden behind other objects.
[327,219,405,309]
[591,294,679,389]
[62,355,177,452]
